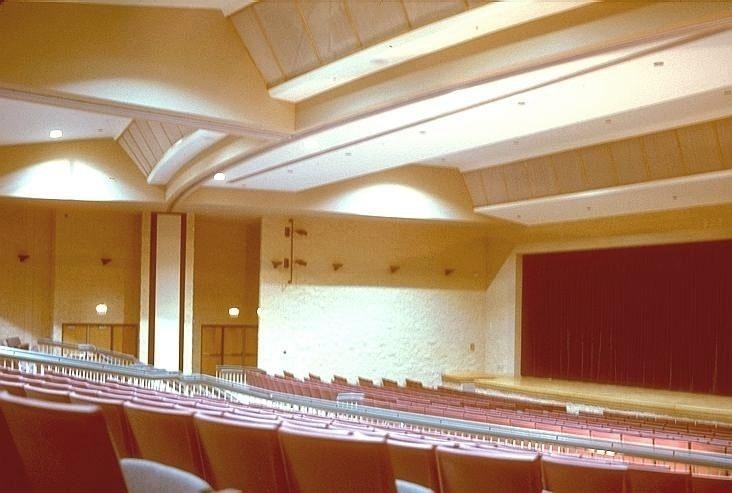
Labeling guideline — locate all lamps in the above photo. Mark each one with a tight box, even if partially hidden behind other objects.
[282,218,309,283]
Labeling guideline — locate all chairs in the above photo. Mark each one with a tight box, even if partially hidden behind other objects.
[0,335,732,491]
